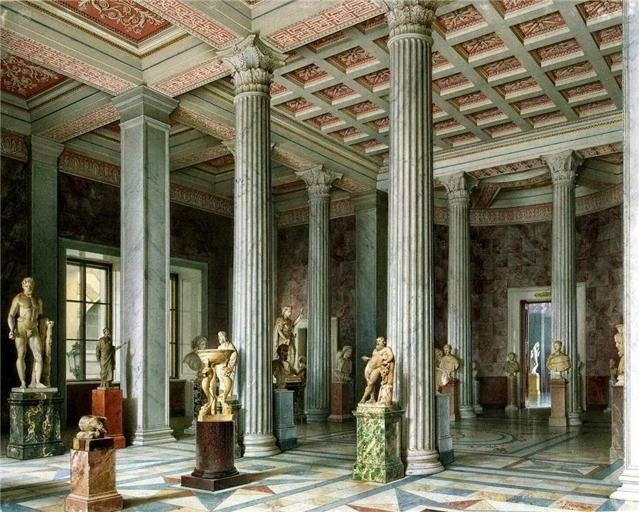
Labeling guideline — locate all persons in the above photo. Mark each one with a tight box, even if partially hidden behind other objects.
[441,344,460,376]
[614,324,625,379]
[545,340,570,372]
[214,330,239,415]
[192,335,220,418]
[358,336,395,403]
[7,276,50,389]
[530,342,540,374]
[272,305,305,367]
[333,345,353,382]
[96,328,114,383]
[270,343,306,388]
[503,351,519,376]
[433,348,449,388]
